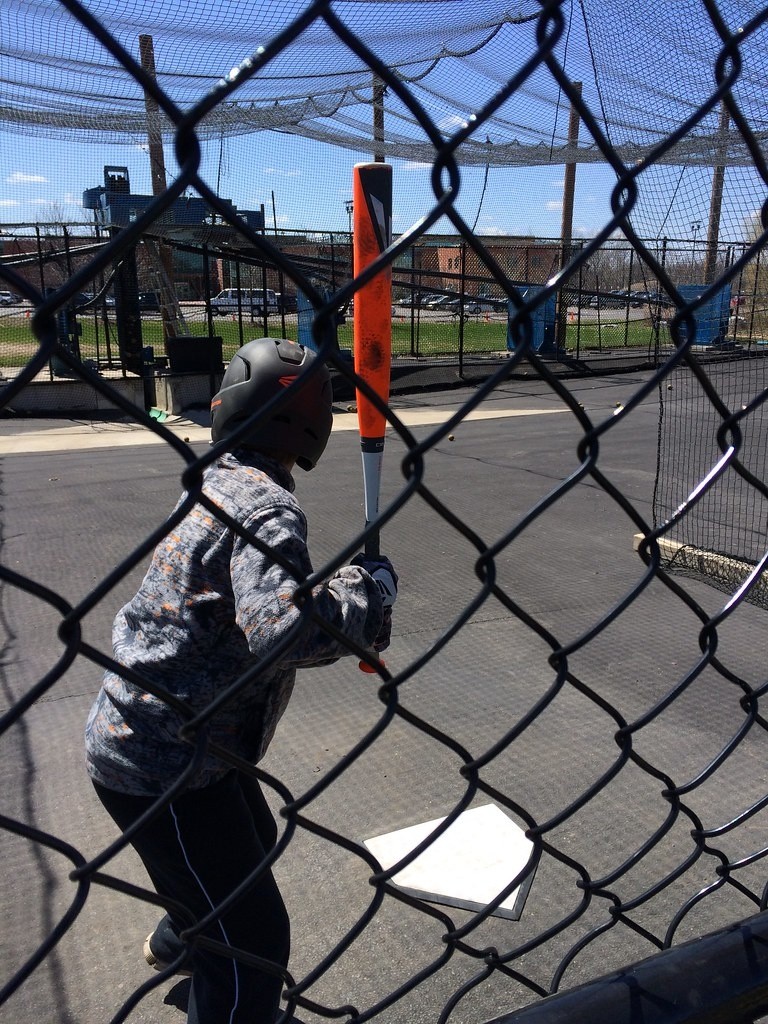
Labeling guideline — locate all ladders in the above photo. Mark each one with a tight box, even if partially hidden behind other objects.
[140,239,190,337]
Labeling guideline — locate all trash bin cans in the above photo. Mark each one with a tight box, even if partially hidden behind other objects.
[163,336,223,373]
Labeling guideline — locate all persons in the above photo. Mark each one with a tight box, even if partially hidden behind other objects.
[81,337,399,1024]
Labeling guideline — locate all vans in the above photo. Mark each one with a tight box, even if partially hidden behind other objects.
[205,287,279,317]
[137,291,161,313]
[0,291,24,306]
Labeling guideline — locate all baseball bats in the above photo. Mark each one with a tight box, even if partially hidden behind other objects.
[348,161,398,676]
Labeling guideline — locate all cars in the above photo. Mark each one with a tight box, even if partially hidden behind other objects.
[399,291,509,314]
[564,289,655,309]
[82,293,116,309]
[339,298,396,317]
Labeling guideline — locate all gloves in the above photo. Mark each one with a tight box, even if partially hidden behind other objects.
[350,552,399,608]
[373,607,393,653]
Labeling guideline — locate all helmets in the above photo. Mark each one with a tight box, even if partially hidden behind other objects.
[210,338,336,471]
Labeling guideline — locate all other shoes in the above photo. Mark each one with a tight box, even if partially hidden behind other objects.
[142,912,189,975]
